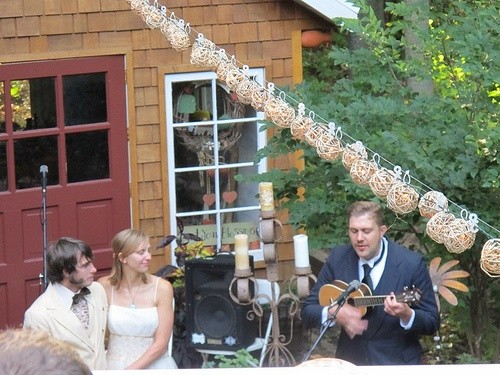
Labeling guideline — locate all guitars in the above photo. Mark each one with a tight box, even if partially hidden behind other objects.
[318,279,423,320]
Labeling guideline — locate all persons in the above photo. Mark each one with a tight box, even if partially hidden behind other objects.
[300,201,440,366]
[0,328,92,375]
[295,357,357,366]
[95,229,179,370]
[22,237,108,371]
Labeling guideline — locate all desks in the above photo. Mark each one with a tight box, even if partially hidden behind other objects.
[194,338,265,368]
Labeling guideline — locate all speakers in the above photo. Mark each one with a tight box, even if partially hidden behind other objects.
[184,254,257,351]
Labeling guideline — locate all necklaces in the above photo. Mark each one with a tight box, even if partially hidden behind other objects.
[124,284,141,309]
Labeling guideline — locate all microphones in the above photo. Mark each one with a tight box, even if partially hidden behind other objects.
[39,165,48,198]
[328,280,360,309]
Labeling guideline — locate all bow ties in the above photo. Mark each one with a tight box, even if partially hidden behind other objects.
[72,287,91,304]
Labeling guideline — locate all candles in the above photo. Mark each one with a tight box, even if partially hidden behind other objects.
[293,234,310,267]
[235,234,249,269]
[258,182,274,211]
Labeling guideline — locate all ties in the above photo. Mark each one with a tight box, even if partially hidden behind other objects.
[361,264,373,292]
